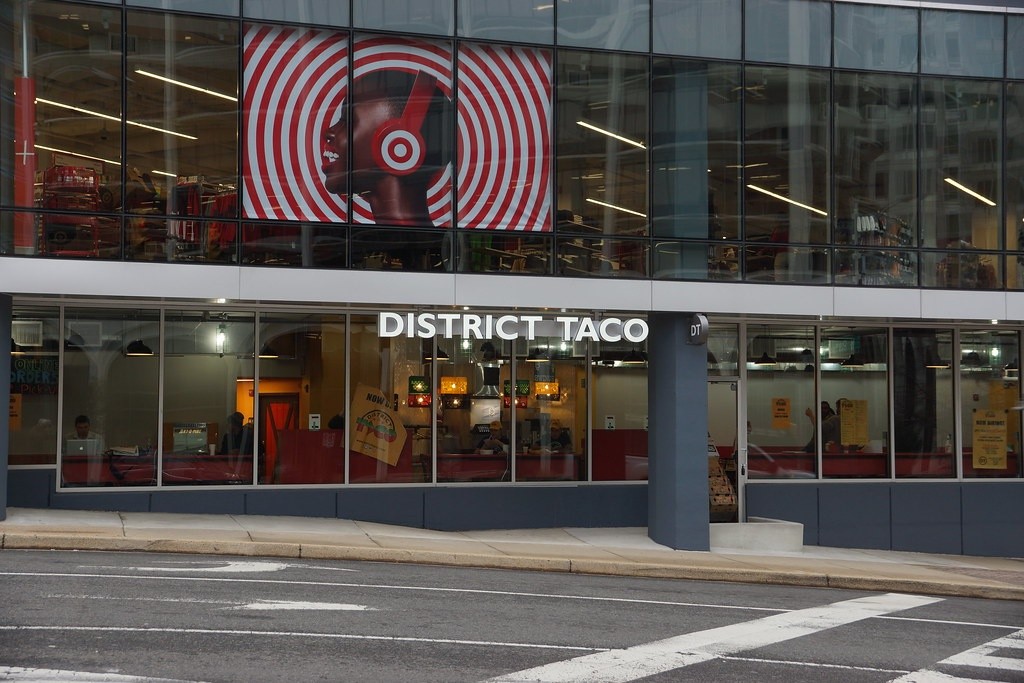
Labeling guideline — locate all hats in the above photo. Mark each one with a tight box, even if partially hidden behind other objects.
[489,421,503,430]
[227,411,244,425]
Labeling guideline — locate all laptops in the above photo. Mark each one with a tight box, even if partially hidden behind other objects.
[66,440,96,456]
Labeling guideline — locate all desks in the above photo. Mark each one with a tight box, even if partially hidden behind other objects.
[62,454,253,482]
[420,453,582,483]
[734,448,1019,479]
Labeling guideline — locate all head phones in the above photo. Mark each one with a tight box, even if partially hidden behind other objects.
[372,68,436,175]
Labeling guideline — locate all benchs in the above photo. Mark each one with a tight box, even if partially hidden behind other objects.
[716,445,805,470]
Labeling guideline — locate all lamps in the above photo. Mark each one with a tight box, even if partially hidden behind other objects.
[425,345,448,361]
[125,310,153,356]
[67,307,85,345]
[479,339,498,360]
[754,323,776,365]
[252,313,278,358]
[925,329,949,368]
[841,326,865,367]
[966,333,981,366]
[622,343,645,364]
[526,337,550,362]
[1003,331,1019,371]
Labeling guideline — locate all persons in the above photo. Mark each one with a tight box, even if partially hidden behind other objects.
[321,70,451,227]
[328,408,345,429]
[474,421,509,454]
[220,411,254,454]
[802,398,864,453]
[730,420,752,469]
[63,415,106,454]
[530,420,573,455]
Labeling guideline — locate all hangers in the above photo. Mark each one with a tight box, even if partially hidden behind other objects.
[210,187,238,198]
[175,175,197,185]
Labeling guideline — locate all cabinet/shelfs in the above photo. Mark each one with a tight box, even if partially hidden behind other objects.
[467,222,645,276]
[34,176,100,252]
